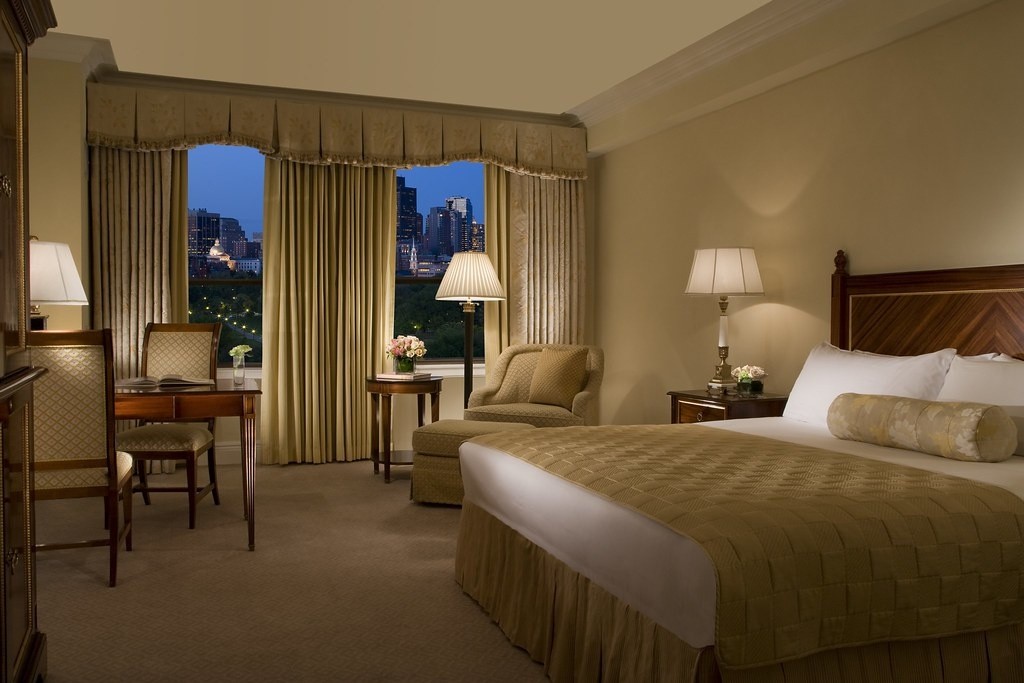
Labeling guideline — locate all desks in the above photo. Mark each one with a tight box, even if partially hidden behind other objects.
[114,378,263,552]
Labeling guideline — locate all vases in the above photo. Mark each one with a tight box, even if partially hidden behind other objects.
[233,355,245,385]
[738,377,764,397]
[395,356,416,375]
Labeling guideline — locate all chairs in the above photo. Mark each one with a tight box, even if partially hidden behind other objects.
[456,250,1024,683]
[27,327,132,587]
[104,322,222,531]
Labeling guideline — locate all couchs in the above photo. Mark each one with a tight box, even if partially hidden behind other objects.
[465,344,604,429]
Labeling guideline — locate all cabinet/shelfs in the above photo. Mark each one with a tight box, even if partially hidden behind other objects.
[0,0,58,683]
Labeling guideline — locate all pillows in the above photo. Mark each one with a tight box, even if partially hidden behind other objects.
[528,346,590,413]
[782,340,1024,463]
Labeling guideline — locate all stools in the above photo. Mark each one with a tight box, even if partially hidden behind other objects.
[409,419,536,507]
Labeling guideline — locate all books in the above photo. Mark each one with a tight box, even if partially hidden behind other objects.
[115,374,216,387]
[377,372,431,380]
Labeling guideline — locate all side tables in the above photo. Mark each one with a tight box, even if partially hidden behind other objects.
[365,376,443,484]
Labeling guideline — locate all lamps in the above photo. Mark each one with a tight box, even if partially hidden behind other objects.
[682,246,765,395]
[434,252,506,410]
[29,235,89,331]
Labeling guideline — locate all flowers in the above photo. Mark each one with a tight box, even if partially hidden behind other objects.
[385,334,427,374]
[731,365,768,380]
[229,345,252,368]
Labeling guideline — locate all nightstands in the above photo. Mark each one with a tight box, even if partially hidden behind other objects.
[666,390,788,424]
